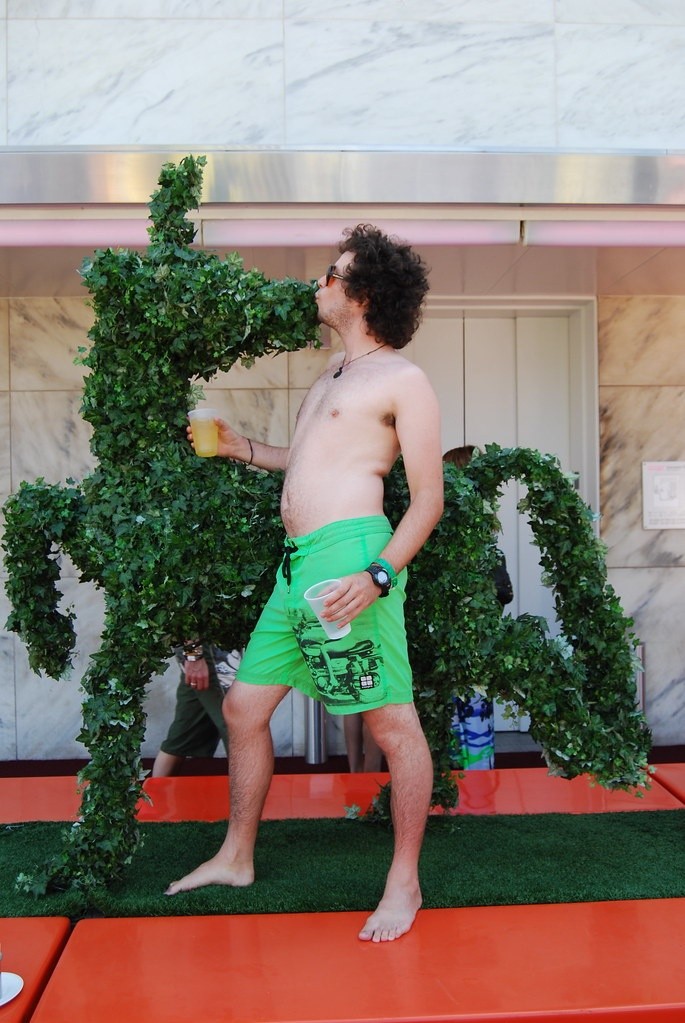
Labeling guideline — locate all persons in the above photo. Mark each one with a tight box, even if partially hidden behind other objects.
[164,224,443,940]
[152,634,243,778]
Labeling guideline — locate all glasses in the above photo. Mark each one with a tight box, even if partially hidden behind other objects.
[326,265,344,286]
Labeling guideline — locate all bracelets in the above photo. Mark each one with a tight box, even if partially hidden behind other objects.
[369,557,397,587]
[244,438,253,466]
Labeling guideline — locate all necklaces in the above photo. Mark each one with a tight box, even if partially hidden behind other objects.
[331,343,387,379]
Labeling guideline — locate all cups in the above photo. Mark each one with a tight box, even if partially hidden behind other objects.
[305,578,352,640]
[188,408,219,457]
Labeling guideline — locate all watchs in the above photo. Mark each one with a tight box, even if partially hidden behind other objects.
[365,565,391,598]
[184,655,202,661]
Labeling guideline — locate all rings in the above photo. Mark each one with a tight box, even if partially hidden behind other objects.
[190,683,196,685]
